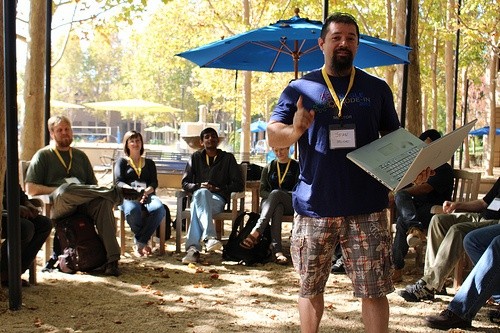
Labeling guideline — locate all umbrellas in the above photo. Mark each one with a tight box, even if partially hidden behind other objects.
[238,118,269,132]
[175,7,413,160]
[86,98,183,130]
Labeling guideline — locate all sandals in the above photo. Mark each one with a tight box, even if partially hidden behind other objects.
[271,252,287,264]
[240,234,258,249]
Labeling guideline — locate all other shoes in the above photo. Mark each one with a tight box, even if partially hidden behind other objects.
[133,246,144,257]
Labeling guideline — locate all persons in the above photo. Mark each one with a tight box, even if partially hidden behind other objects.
[0,173,53,288]
[114,131,166,258]
[182,128,242,264]
[268,13,435,333]
[388,130,454,282]
[399,176,500,301]
[240,146,299,263]
[25,115,139,275]
[427,224,500,329]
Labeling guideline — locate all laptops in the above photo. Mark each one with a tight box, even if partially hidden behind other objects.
[346,118,479,193]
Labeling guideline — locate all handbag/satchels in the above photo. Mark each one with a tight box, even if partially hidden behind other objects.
[57,235,107,274]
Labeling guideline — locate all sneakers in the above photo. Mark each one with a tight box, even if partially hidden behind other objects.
[398,286,434,301]
[205,238,222,251]
[182,246,199,264]
[406,279,426,289]
[391,269,403,283]
[331,257,346,273]
[426,309,472,331]
[487,309,500,327]
[407,228,427,246]
